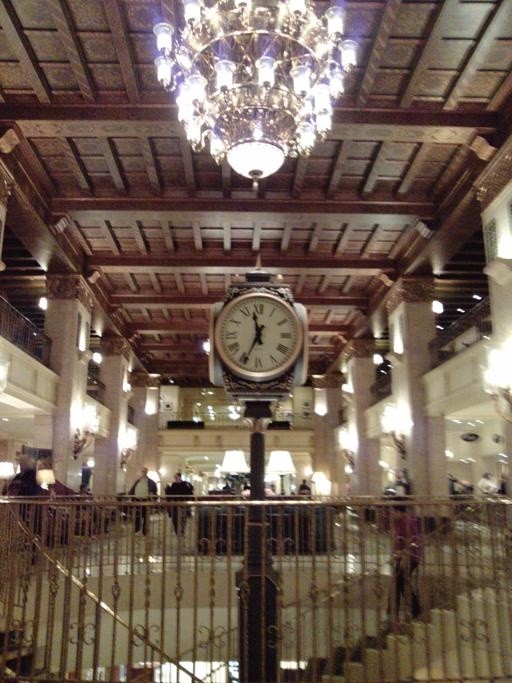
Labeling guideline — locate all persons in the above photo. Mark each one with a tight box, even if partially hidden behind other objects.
[3,452,37,565]
[165,484,172,517]
[394,469,409,496]
[298,479,311,497]
[448,471,509,497]
[383,498,421,620]
[127,467,158,536]
[167,472,192,535]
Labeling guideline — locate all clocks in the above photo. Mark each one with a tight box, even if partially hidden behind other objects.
[213,290,305,384]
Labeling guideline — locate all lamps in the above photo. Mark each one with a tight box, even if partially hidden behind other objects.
[381,404,409,459]
[339,430,355,474]
[152,0,359,191]
[74,406,100,459]
[266,450,297,495]
[119,428,137,473]
[220,450,250,495]
[485,338,512,423]
